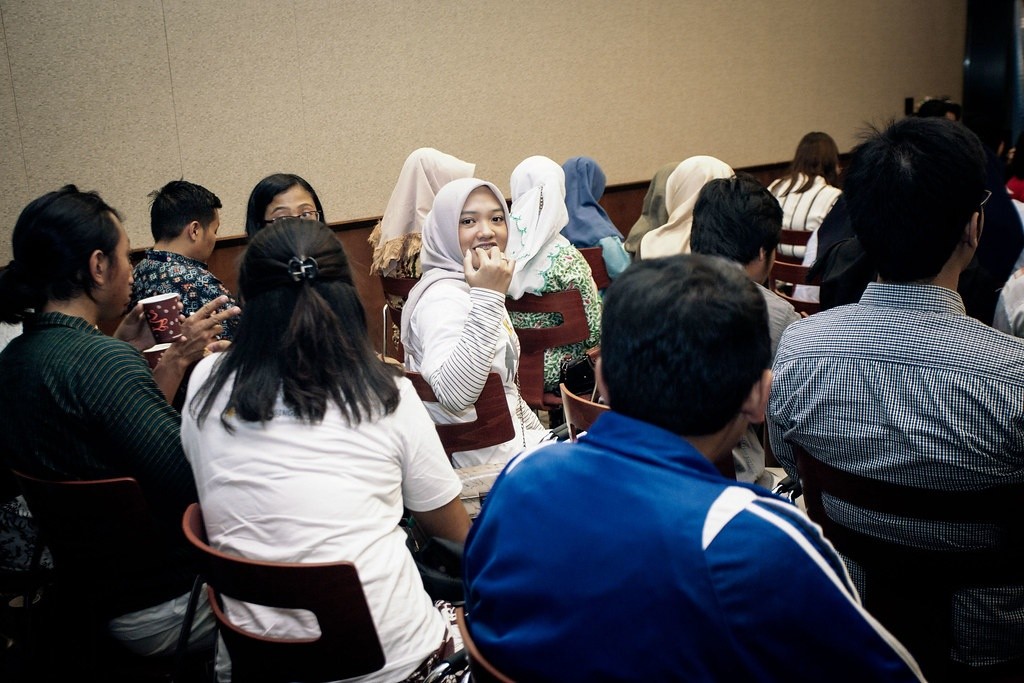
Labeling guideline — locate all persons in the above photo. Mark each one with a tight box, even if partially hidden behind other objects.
[180,216,474,683]
[0,173,326,657]
[765,115,1024,683]
[462,254,929,683]
[367,100,1024,520]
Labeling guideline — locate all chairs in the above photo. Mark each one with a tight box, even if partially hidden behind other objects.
[10,228,1024,682]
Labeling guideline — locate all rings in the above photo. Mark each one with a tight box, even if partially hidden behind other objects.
[501,258,509,264]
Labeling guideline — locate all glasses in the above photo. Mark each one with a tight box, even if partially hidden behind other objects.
[980,190,992,208]
[265,210,321,224]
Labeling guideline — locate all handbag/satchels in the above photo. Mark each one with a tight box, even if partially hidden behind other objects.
[553,354,598,397]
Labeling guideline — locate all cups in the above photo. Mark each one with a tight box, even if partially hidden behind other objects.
[138,292,183,343]
